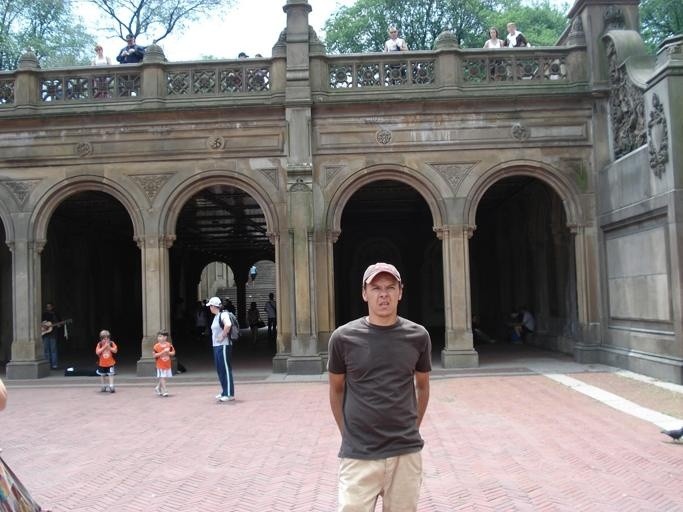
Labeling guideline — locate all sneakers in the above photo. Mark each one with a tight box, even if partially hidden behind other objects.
[216,395,235,401]
[102,387,106,392]
[154,387,168,396]
[110,388,115,392]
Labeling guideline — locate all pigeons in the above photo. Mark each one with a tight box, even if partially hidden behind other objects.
[661,426,683,444]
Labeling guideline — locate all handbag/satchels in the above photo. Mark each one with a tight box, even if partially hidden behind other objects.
[257,321,265,327]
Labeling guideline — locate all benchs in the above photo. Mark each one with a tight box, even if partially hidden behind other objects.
[524,315,575,356]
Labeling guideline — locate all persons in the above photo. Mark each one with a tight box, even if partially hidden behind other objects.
[250,264,257,287]
[195,293,276,347]
[472,315,481,350]
[153,328,175,396]
[206,297,236,401]
[41,302,64,369]
[96,330,118,392]
[325,262,431,512]
[0,378,7,411]
[511,307,536,344]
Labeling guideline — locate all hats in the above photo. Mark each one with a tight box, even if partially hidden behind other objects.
[362,262,402,288]
[205,297,221,307]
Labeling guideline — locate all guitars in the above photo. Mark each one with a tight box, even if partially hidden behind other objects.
[42,320,72,336]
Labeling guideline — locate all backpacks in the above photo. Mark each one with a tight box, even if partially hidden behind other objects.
[228,312,241,342]
